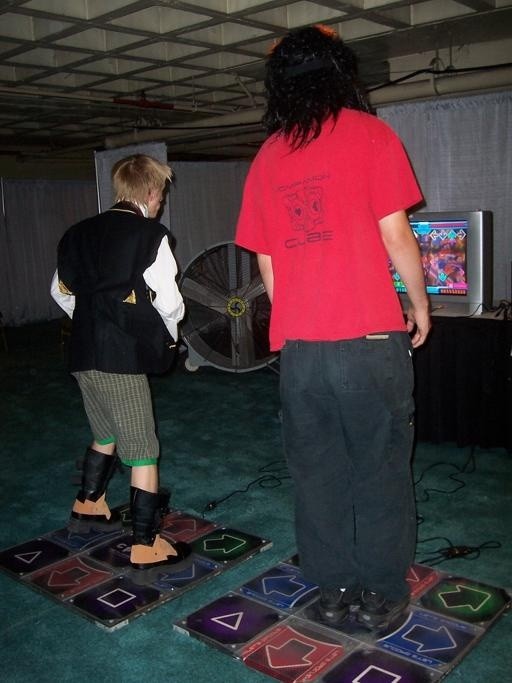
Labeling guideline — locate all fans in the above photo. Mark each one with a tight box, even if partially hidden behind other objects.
[175,238,282,375]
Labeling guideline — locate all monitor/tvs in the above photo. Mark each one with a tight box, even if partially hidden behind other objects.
[387,209,493,316]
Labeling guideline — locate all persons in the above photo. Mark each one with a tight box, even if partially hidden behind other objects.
[49,153,193,570]
[233,19,435,625]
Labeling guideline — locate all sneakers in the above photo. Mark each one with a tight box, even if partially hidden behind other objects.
[316,585,411,630]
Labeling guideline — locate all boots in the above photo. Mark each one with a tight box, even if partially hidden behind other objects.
[68,445,122,534]
[129,485,191,570]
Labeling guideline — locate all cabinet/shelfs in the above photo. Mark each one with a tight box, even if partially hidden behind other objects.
[402,306,512,448]
